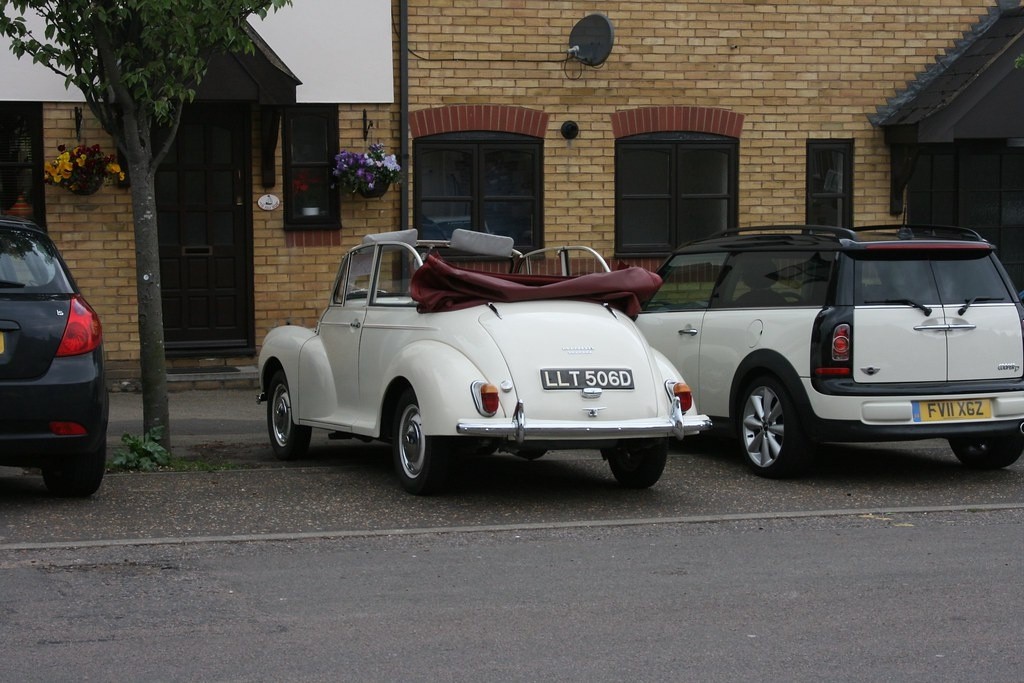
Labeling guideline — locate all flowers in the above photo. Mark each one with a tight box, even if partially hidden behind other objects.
[291,168,321,207]
[42,143,125,192]
[330,142,404,195]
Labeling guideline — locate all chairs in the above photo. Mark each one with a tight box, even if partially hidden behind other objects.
[732,259,788,306]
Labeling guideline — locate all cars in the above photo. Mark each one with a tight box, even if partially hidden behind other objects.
[252,229,713,493]
[623,220,1024,482]
[0,216,108,503]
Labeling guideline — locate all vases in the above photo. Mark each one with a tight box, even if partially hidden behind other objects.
[302,207,320,216]
[356,179,390,198]
